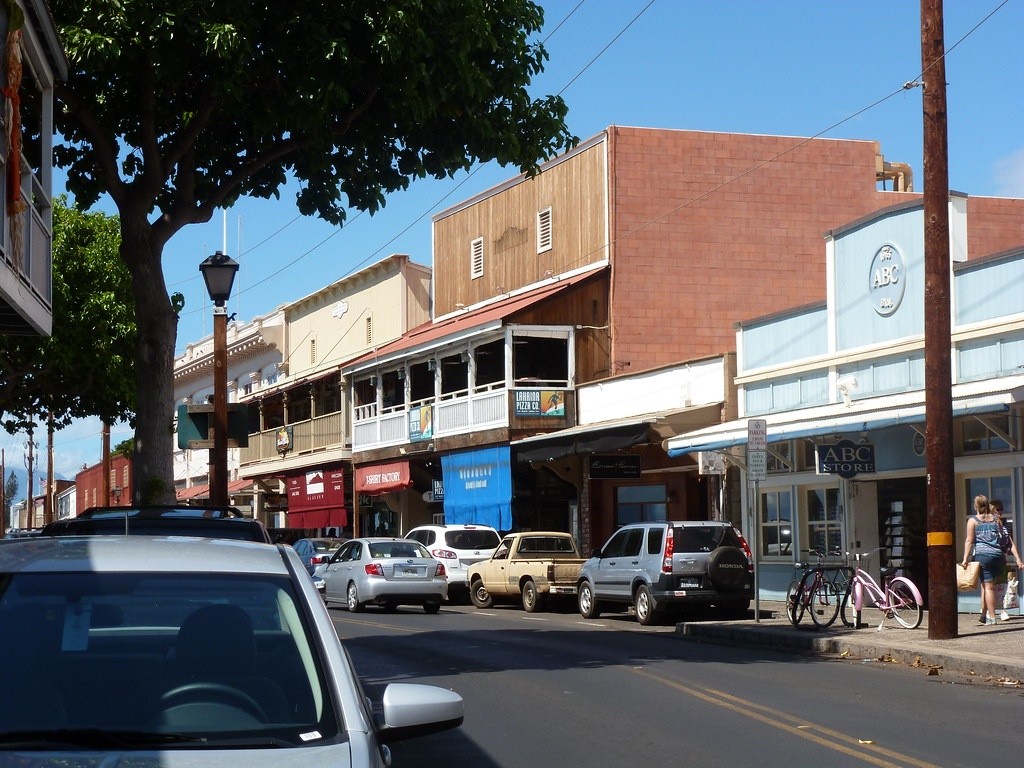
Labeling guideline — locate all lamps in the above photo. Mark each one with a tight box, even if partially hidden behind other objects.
[459,350,469,363]
[370,377,377,386]
[397,368,406,380]
[428,358,436,371]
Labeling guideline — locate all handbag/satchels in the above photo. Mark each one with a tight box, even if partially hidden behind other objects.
[997,523,1012,552]
[955,562,980,591]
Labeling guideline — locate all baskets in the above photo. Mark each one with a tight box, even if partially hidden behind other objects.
[847,559,868,576]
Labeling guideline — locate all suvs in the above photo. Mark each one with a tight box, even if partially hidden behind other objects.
[762,518,794,555]
[41,504,282,631]
[405,525,507,592]
[5,529,51,555]
[576,520,754,627]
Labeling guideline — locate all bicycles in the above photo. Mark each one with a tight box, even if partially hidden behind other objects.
[837,548,924,632]
[786,544,841,627]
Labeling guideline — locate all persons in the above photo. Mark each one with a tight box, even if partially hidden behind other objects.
[328,528,337,537]
[547,391,562,411]
[962,495,1024,625]
[423,411,431,436]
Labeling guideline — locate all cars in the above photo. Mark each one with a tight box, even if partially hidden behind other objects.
[291,536,352,576]
[0,537,465,768]
[309,536,449,614]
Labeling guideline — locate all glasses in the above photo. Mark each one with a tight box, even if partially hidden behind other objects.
[993,508,1003,512]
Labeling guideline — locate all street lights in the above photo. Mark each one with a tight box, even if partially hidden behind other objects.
[198,251,240,509]
[115,486,122,503]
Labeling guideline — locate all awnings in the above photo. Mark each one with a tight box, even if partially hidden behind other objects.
[341,267,606,377]
[176,474,269,500]
[662,374,1024,454]
[510,402,722,483]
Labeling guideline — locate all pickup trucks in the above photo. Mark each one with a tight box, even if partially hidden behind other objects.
[466,532,591,611]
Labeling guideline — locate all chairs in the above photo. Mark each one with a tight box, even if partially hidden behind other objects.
[0,622,66,727]
[130,604,291,725]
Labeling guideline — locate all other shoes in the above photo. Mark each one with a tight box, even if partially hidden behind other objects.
[1001,612,1010,621]
[980,609,990,617]
[979,616,986,623]
[988,618,997,625]
